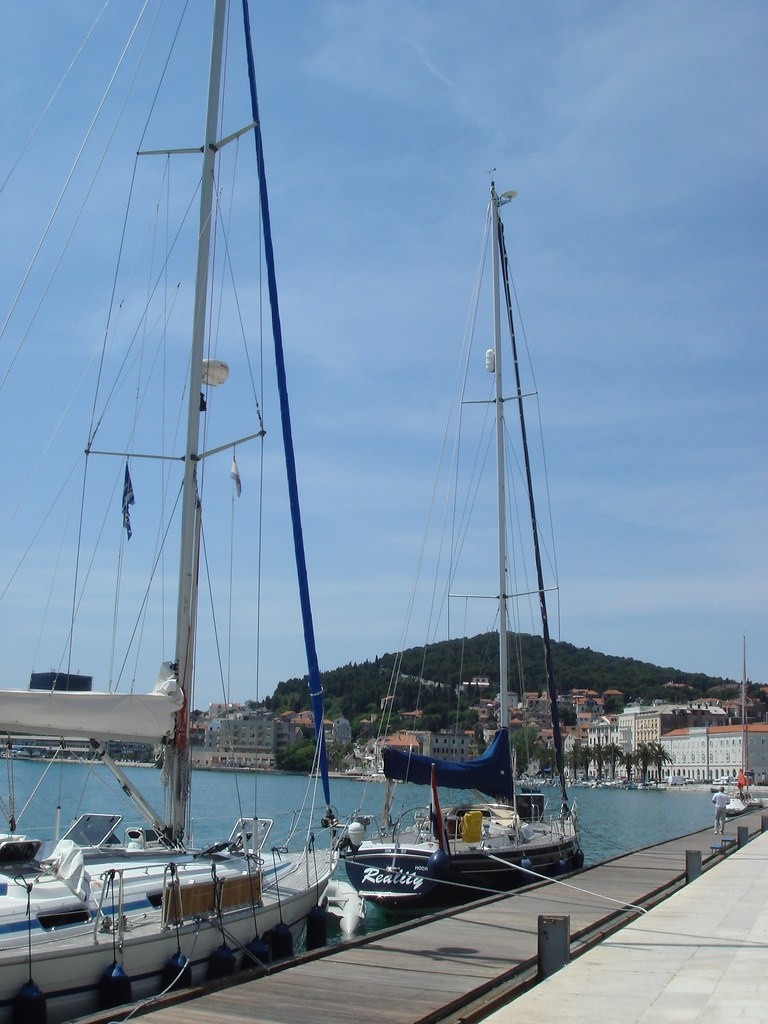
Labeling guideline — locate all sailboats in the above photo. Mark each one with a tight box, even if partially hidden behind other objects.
[342,169,585,918]
[0,2,346,1021]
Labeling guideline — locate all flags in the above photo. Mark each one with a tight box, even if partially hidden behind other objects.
[428,768,451,856]
[122,463,136,541]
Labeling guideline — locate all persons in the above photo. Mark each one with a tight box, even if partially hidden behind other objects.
[711,787,730,834]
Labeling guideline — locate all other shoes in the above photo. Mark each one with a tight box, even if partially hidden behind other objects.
[720,832,723,834]
[714,831,718,834]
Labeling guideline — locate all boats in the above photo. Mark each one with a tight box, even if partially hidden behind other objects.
[721,788,763,817]
